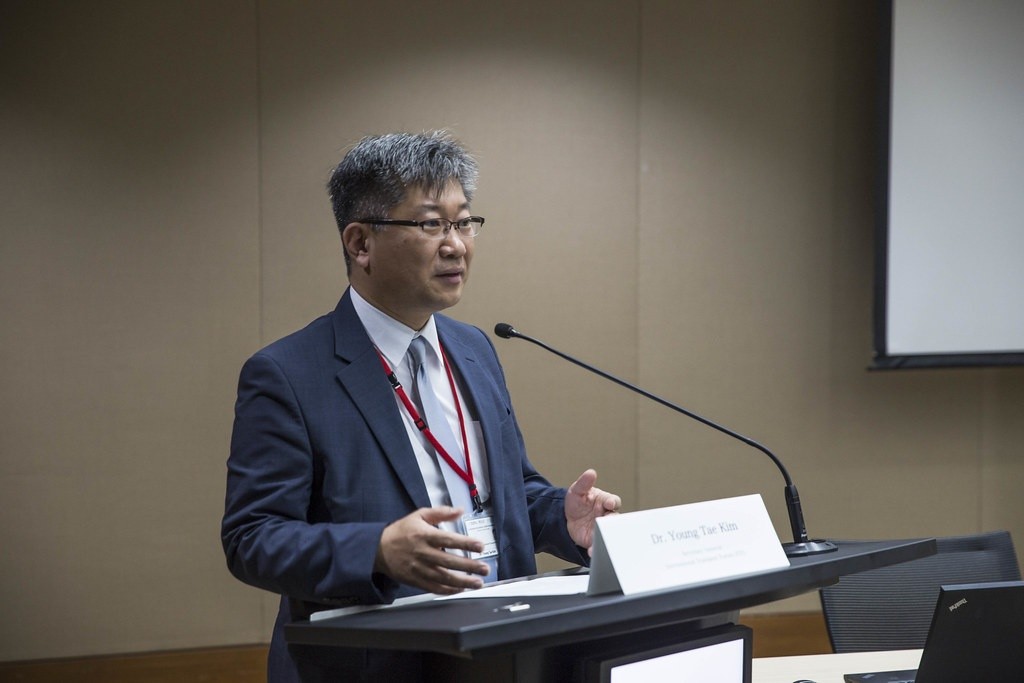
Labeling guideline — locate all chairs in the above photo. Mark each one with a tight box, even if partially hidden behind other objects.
[819,532,1022,654]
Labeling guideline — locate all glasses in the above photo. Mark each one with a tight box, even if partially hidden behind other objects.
[359,216,485,240]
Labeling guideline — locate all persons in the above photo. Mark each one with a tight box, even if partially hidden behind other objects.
[221,129,623,683]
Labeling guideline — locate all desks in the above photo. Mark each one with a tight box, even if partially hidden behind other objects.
[752,649,924,683]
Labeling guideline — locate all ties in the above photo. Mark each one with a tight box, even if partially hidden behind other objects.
[409,336,498,584]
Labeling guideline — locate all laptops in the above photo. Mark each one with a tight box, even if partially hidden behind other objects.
[844,582,1024,683]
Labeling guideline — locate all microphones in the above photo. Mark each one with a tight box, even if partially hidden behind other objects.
[493,322,839,557]
